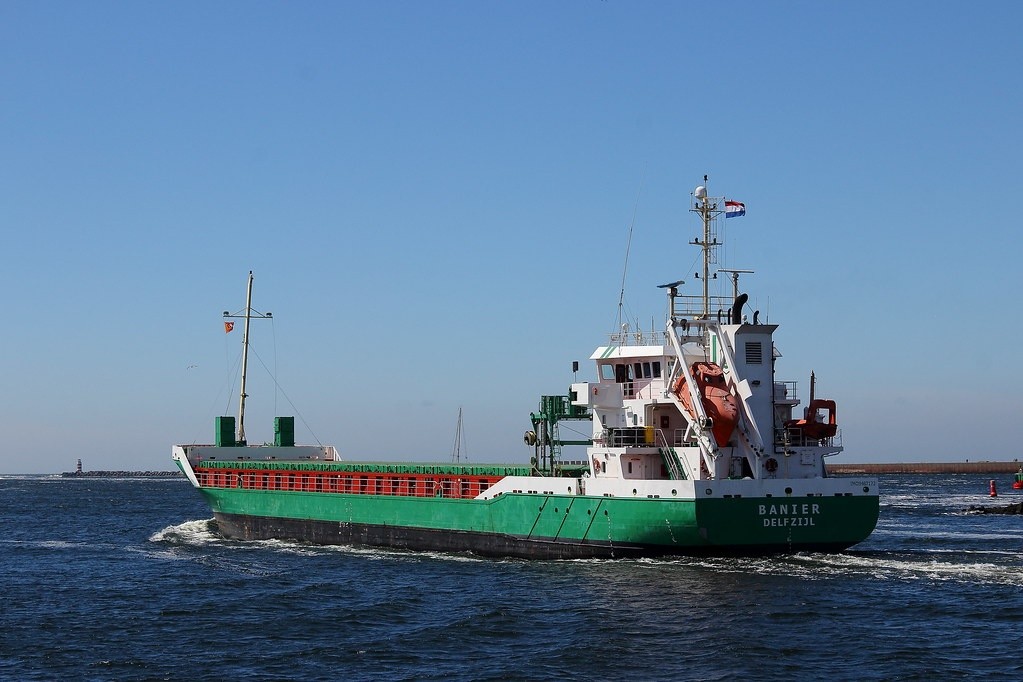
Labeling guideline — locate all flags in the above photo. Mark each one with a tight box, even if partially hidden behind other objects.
[723,199,746,218]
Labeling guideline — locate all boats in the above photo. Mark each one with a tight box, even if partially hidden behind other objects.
[172,175,880,560]
[669,362,740,448]
